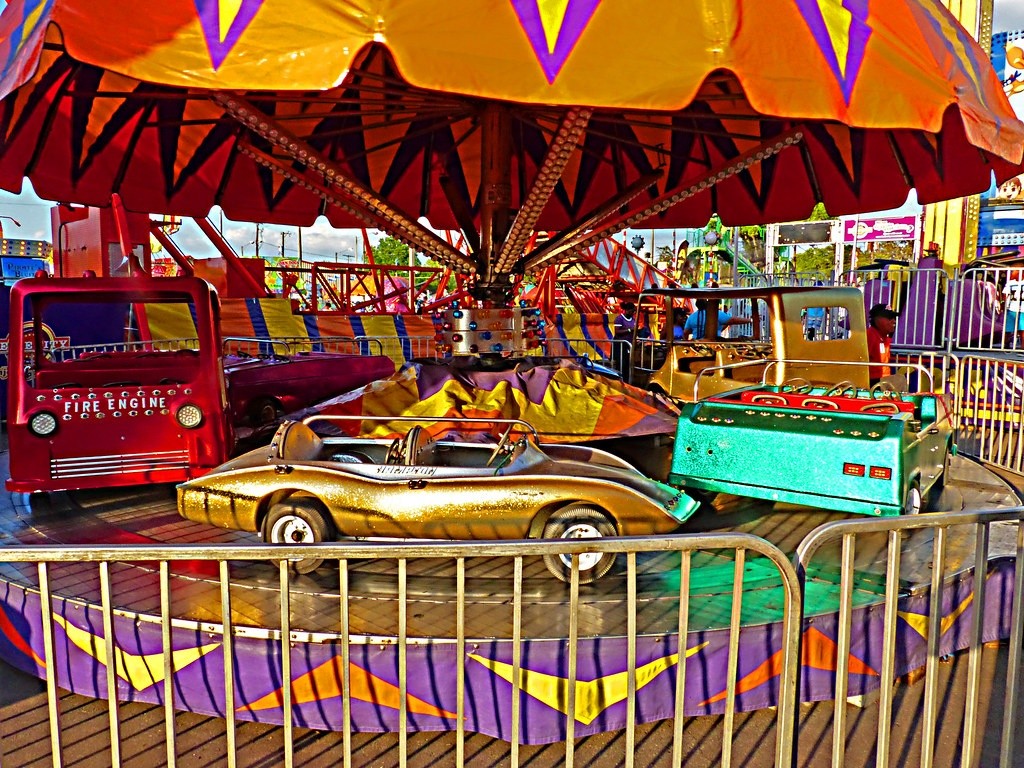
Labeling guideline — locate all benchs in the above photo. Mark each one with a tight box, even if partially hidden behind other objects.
[42,357,175,370]
[405,425,444,466]
[278,420,376,465]
[35,367,178,388]
[296,351,346,357]
[741,391,915,415]
[79,351,177,360]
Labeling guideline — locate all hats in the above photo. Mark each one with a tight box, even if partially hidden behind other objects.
[868,303,900,318]
[925,241,940,252]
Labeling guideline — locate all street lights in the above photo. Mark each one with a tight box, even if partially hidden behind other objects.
[241,241,254,255]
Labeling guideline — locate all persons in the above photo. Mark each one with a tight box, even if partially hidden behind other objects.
[865,303,899,390]
[322,286,450,313]
[1001,279,1024,357]
[613,240,827,371]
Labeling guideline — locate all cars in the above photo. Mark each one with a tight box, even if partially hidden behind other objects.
[176,413,703,588]
